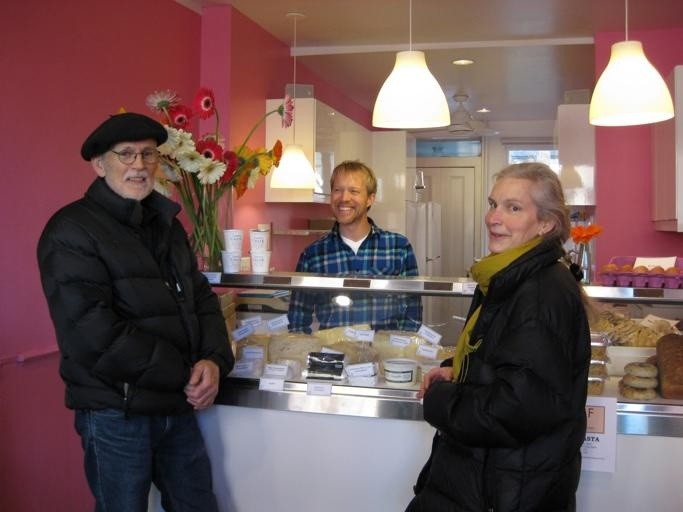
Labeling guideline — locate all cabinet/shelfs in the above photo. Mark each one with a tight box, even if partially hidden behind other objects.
[552,103,595,208]
[652,65,683,236]
[368,131,416,267]
[199,266,683,512]
[264,98,373,207]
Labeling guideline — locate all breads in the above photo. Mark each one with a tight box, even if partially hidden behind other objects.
[600,263,682,401]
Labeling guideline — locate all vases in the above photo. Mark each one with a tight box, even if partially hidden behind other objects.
[574,241,593,285]
[176,172,233,273]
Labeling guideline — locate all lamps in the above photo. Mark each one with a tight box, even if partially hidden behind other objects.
[588,1,673,129]
[370,0,450,131]
[269,12,320,191]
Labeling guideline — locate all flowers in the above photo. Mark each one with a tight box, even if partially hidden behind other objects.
[570,224,601,245]
[102,85,295,271]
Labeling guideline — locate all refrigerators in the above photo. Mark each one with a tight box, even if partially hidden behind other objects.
[415,201,445,326]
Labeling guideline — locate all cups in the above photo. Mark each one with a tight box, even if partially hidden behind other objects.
[249,229,269,251]
[222,229,243,252]
[250,250,271,274]
[220,250,242,274]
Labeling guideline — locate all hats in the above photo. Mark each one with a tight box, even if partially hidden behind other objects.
[80,112,169,162]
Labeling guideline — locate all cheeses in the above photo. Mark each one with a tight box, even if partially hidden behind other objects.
[236,323,455,383]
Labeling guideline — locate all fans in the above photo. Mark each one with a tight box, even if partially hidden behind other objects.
[425,93,503,137]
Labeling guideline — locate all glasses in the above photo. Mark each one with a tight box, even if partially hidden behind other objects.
[110,147,163,165]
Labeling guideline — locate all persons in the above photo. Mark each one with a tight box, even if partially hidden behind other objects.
[286,160,423,334]
[407,164,592,512]
[36,113,236,512]
